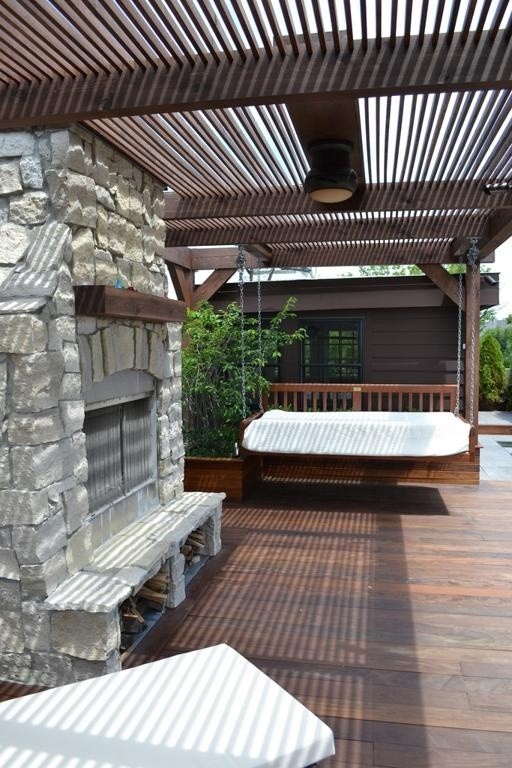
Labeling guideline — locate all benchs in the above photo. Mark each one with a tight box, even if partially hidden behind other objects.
[44,490,228,666]
[240,382,477,465]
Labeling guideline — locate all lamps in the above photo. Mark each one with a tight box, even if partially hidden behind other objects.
[304,137,358,203]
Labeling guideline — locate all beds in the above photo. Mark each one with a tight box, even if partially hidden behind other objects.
[0,643,336,768]
